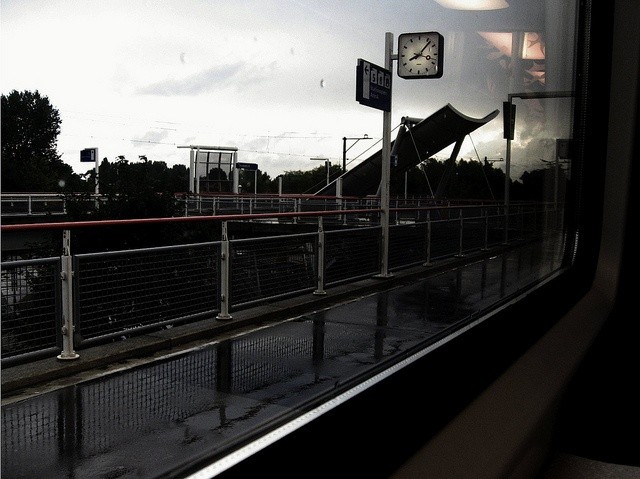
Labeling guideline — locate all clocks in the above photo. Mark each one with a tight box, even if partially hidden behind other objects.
[398,32,444,78]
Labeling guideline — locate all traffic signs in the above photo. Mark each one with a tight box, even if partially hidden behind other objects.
[355,58,392,112]
[80,149,95,163]
